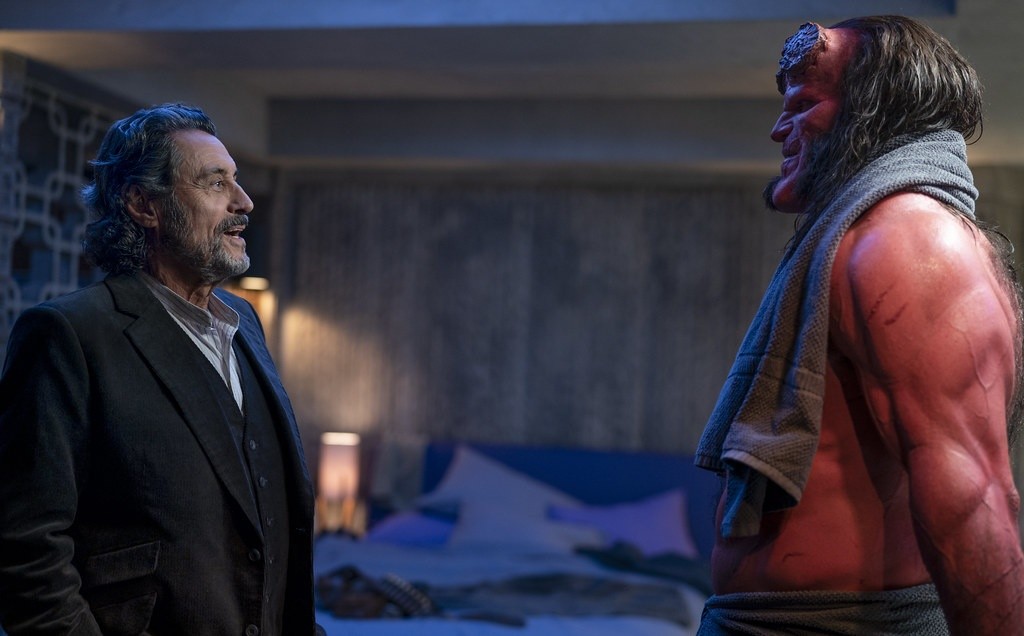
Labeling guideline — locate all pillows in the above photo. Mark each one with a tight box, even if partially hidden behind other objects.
[443,521,606,560]
[548,480,700,561]
[421,447,582,522]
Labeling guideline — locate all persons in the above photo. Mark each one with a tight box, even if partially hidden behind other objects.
[696,14,1023,636]
[0,104,321,636]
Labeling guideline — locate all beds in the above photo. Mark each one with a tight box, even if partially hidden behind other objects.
[305,439,723,636]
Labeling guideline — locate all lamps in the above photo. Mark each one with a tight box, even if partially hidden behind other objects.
[315,431,368,537]
